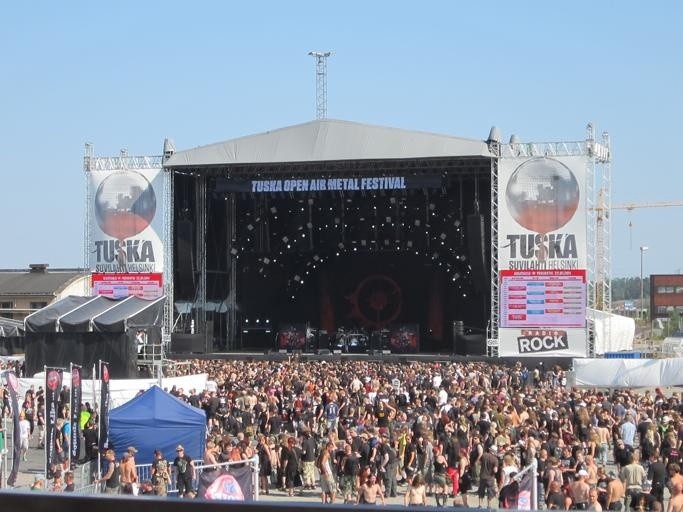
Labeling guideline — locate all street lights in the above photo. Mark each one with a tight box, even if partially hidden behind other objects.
[640,246,649,320]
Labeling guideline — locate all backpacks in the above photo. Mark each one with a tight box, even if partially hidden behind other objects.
[314,450,323,468]
[343,454,359,475]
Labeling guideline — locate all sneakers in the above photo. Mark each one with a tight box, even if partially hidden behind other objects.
[19,456,27,461]
[463,503,492,512]
[289,485,316,497]
[384,494,397,498]
[38,441,45,449]
[259,484,286,495]
[426,489,454,508]
[398,477,409,487]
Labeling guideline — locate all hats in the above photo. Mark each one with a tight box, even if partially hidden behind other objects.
[123,446,138,457]
[489,441,503,453]
[270,388,276,393]
[662,416,669,423]
[35,474,44,481]
[207,442,215,448]
[176,445,183,451]
[358,433,378,447]
[153,449,160,454]
[574,469,588,479]
[20,410,25,417]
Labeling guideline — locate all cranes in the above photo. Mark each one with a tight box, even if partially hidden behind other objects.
[588,187,683,311]
